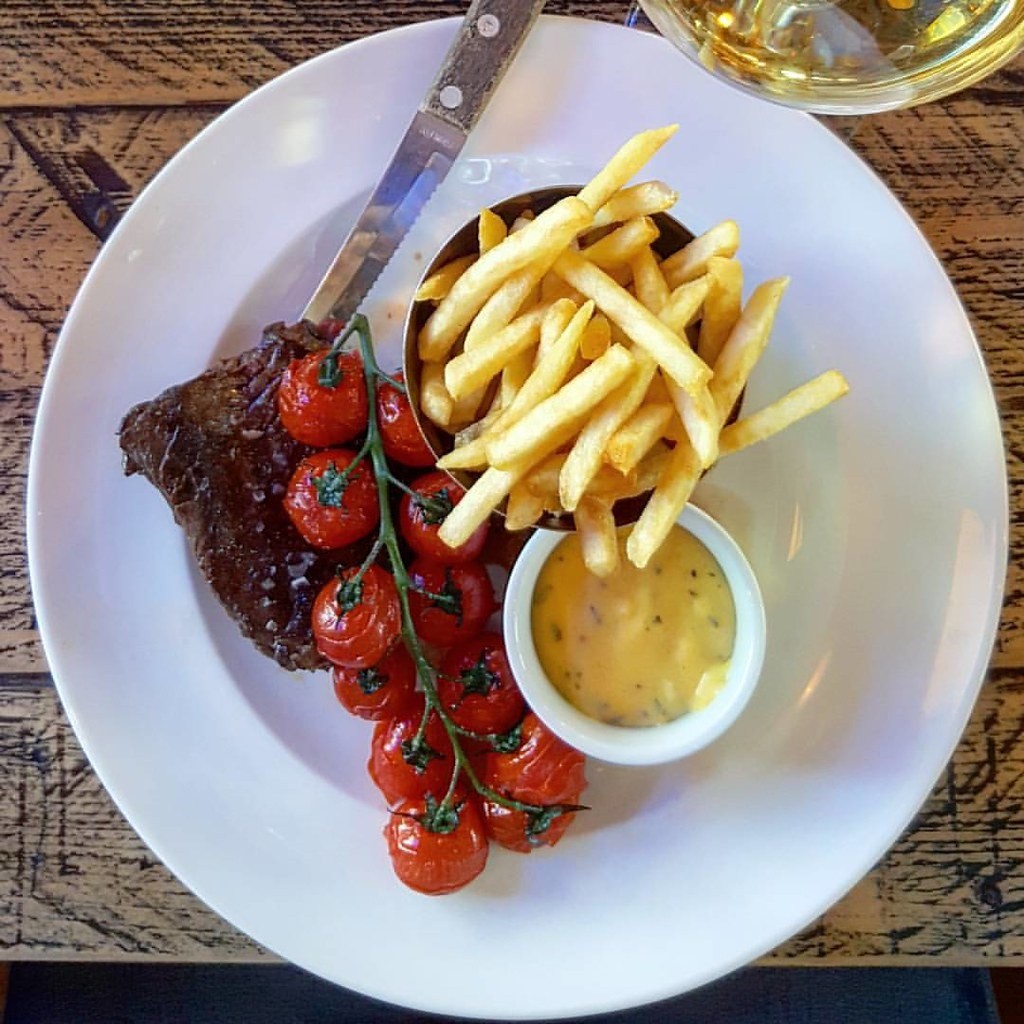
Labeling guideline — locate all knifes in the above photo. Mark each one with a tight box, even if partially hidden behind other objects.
[297,0,548,347]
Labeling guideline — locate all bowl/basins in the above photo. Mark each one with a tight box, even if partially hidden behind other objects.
[402,183,748,532]
[503,502,767,765]
[637,0,1024,116]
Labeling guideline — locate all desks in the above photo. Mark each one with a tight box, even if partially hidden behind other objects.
[0,0,1024,965]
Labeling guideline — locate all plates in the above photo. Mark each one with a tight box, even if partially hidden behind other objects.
[26,13,1010,1020]
